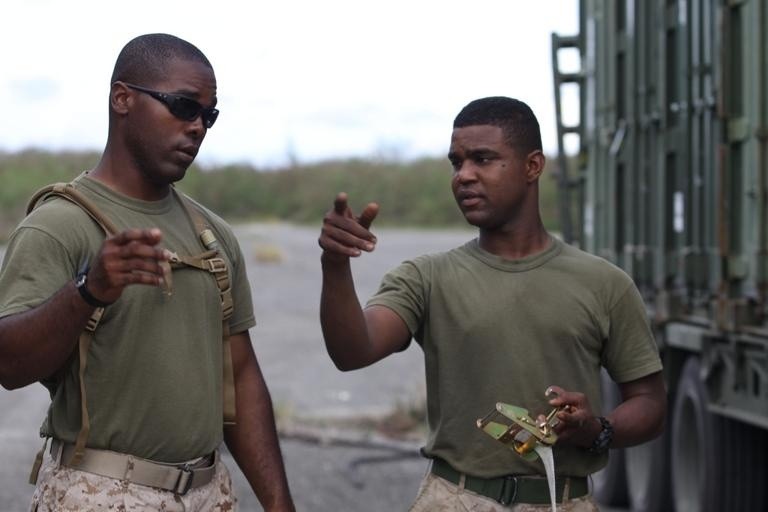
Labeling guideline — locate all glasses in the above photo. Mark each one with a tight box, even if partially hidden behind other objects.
[127,83,219,129]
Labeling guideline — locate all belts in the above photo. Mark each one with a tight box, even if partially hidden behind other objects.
[50,439,219,494]
[432,459,589,506]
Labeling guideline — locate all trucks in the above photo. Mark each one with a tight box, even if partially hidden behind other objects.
[551,2,766,507]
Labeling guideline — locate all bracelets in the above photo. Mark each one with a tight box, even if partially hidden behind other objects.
[74,265,117,308]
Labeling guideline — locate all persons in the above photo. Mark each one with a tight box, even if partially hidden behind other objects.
[1,32,301,511]
[319,94,671,512]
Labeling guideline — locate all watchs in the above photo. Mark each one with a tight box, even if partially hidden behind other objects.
[586,414,614,456]
